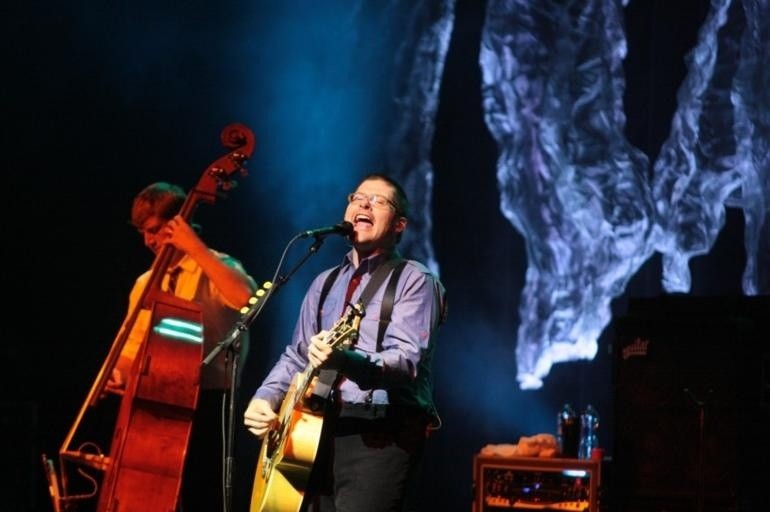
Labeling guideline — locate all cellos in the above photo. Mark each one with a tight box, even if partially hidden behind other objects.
[99,125,255,512]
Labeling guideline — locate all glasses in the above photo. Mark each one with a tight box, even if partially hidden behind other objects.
[348,192,399,214]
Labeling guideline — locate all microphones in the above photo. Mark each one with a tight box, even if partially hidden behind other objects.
[300,220,354,238]
[62,449,110,472]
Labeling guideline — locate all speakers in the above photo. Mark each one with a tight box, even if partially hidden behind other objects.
[609,294,769,511]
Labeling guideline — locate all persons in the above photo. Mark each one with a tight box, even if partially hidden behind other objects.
[91,182,258,511]
[241,170,447,512]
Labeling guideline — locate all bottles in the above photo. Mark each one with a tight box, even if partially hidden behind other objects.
[555,402,600,461]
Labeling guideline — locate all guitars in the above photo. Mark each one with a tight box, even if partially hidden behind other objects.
[249,305,364,510]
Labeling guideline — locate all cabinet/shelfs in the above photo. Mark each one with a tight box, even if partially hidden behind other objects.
[471,446,604,512]
